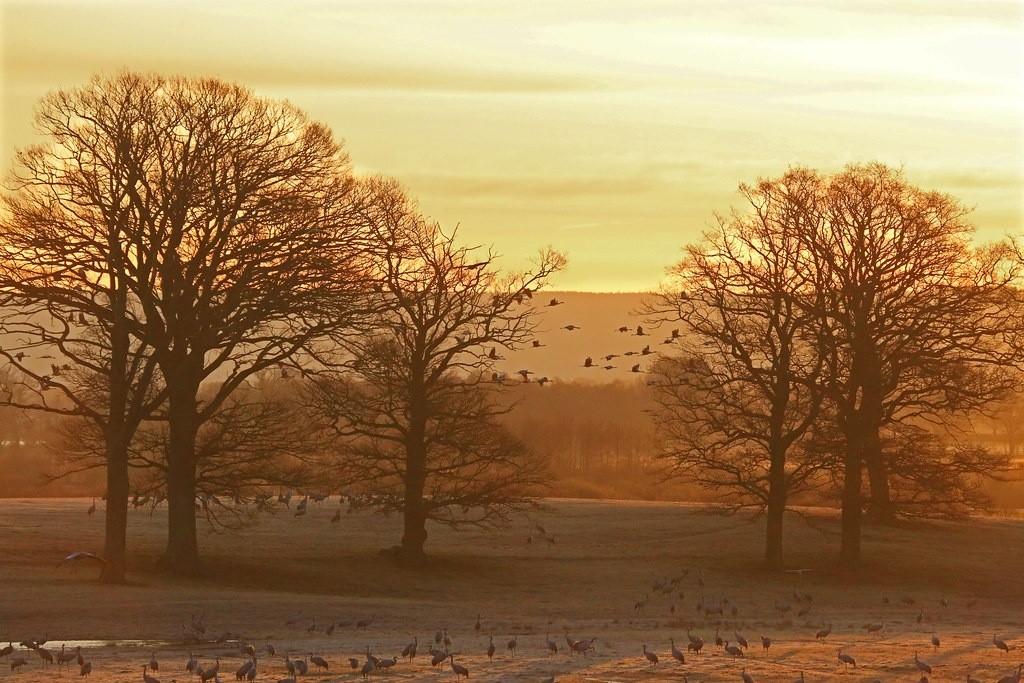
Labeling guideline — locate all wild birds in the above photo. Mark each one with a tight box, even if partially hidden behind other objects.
[1,260,695,389]
[0,567,1023,681]
[527,524,554,546]
[89,480,404,520]
